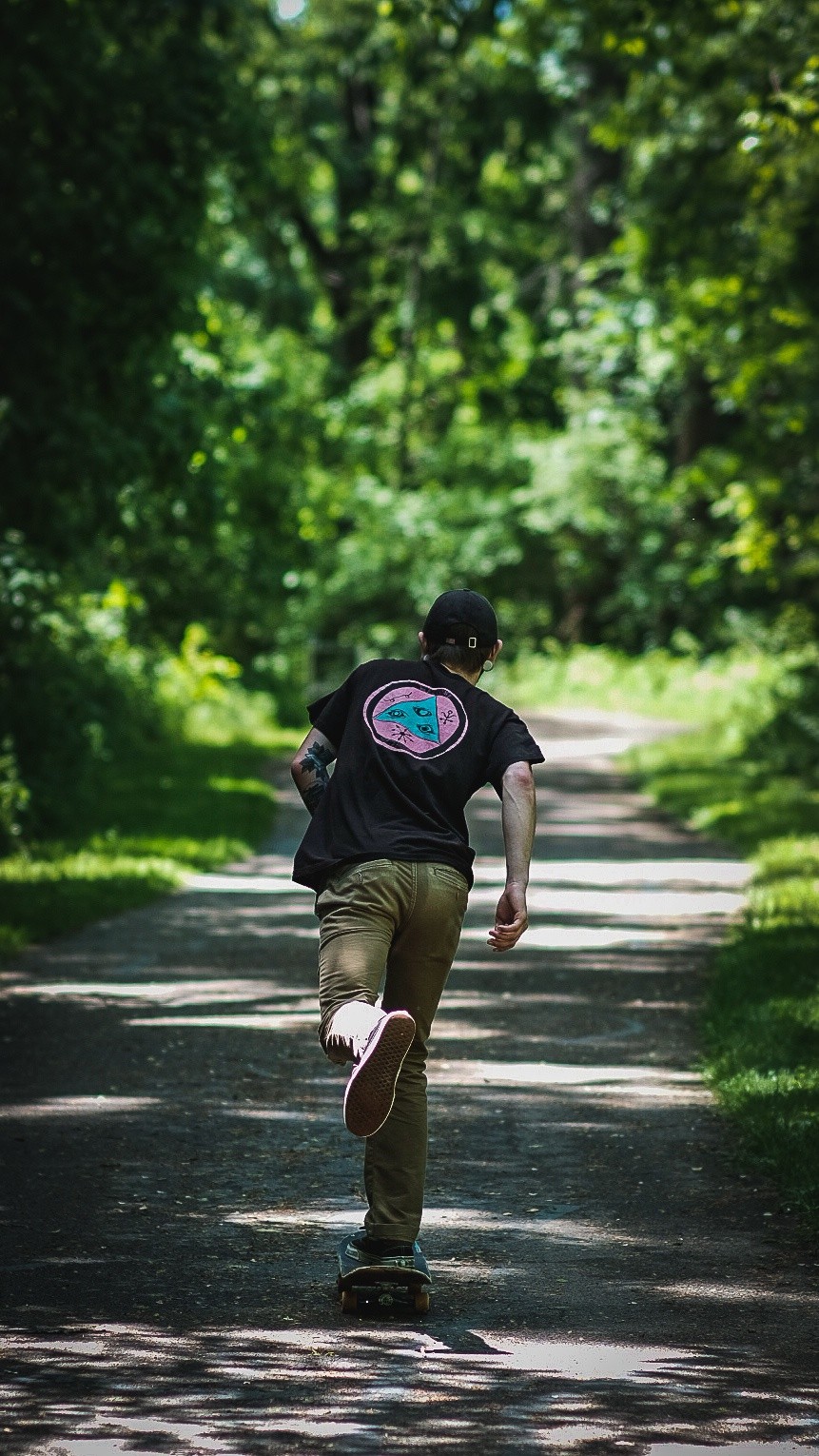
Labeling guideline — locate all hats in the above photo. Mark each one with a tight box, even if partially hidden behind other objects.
[424,588,498,649]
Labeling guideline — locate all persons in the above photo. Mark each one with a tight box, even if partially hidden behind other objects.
[288,589,546,1270]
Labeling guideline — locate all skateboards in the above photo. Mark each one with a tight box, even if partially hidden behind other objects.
[338,1227,432,1310]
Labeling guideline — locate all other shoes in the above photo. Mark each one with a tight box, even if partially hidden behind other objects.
[344,1237,415,1272]
[343,1010,415,1138]
